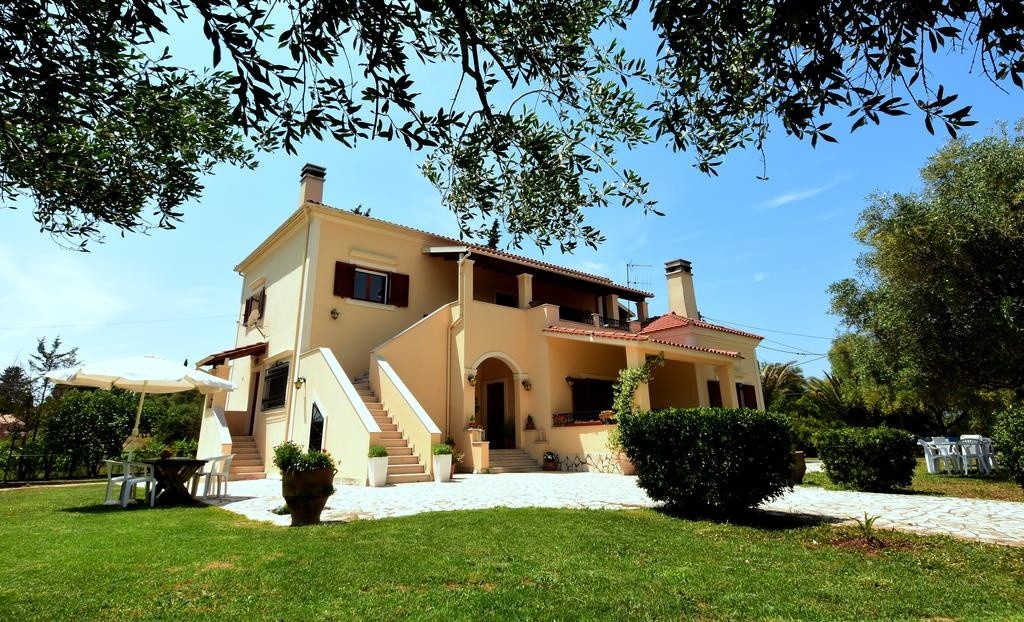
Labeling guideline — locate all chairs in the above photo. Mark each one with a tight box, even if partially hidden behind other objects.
[916,435,999,476]
[102,453,235,509]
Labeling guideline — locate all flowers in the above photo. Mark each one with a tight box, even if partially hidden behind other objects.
[272,442,333,477]
[543,451,556,461]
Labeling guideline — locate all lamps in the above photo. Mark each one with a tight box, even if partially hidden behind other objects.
[294,377,306,389]
[469,376,477,385]
[522,381,531,391]
[566,376,574,386]
[331,309,339,319]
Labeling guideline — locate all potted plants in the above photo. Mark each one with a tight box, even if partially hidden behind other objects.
[789,446,805,484]
[449,448,466,478]
[367,444,388,487]
[468,415,476,427]
[609,428,638,475]
[526,415,534,429]
[432,444,452,481]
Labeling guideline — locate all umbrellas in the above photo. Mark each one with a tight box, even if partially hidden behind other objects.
[44,353,239,461]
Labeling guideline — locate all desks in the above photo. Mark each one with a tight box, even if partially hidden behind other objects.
[916,440,993,473]
[143,456,208,505]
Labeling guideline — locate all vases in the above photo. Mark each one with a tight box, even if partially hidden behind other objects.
[543,460,556,470]
[281,467,333,526]
